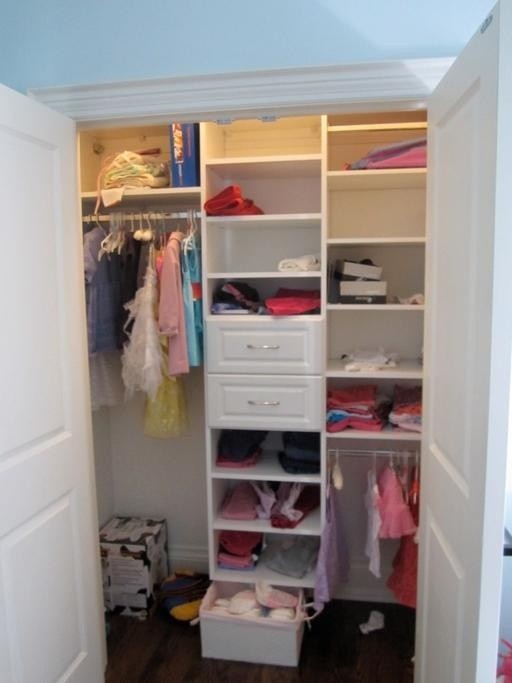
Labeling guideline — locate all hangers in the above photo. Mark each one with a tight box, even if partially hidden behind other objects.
[325,447,419,504]
[87,208,198,261]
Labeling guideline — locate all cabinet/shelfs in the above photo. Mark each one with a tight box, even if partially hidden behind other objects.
[77,99,429,589]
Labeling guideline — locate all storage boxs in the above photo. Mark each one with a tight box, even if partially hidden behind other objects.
[98,516,171,620]
[199,581,307,668]
